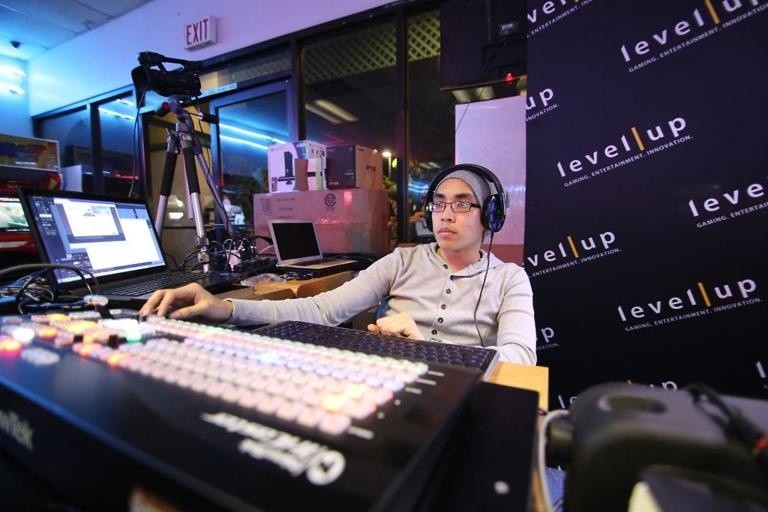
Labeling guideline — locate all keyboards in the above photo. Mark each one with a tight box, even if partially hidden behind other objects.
[253,320,498,382]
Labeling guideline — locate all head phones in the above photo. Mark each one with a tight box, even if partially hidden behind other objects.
[425,163,510,232]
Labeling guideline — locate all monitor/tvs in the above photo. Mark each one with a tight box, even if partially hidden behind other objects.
[0,195,31,232]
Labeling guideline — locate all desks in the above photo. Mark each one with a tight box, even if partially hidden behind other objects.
[130,271,549,512]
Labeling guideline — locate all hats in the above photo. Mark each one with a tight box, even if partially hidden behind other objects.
[434,169,491,212]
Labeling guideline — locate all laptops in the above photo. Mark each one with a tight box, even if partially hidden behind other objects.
[17,185,240,310]
[268,219,357,277]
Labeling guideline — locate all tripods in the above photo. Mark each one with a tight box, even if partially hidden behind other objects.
[154,95,238,272]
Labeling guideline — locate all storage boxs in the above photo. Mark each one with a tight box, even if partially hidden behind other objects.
[295,158,321,191]
[253,189,390,259]
[268,140,327,194]
[327,144,383,189]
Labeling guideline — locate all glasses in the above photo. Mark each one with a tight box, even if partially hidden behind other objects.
[428,200,480,213]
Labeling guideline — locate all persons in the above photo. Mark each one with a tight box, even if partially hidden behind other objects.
[131,164,539,365]
